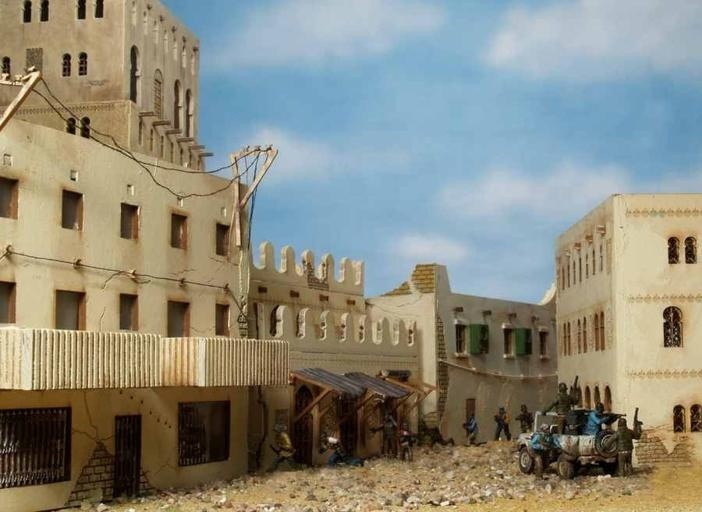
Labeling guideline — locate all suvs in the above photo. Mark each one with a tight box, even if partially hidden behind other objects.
[519,404,630,481]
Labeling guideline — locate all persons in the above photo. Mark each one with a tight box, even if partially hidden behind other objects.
[493,406,512,441]
[267,424,305,472]
[462,413,479,447]
[515,405,534,433]
[397,423,414,463]
[417,420,456,448]
[370,412,397,457]
[316,433,367,468]
[530,382,643,480]
